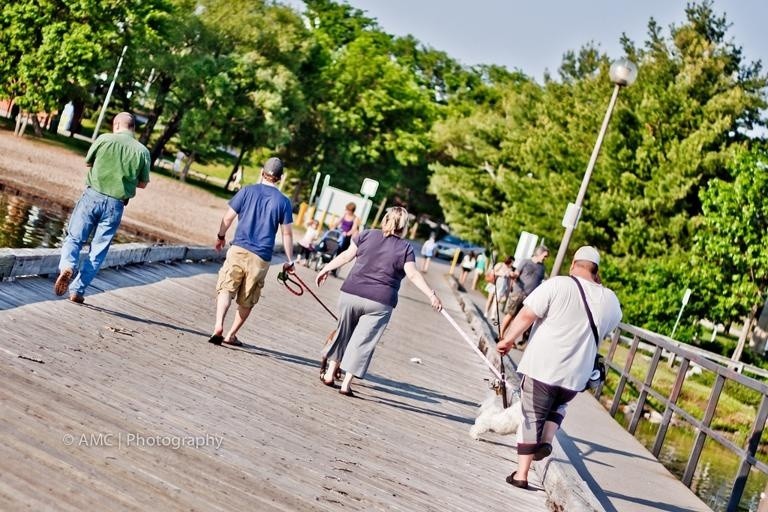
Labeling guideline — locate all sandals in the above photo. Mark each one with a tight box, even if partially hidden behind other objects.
[339,385,355,397]
[320,372,335,387]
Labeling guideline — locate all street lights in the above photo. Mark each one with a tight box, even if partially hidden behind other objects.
[548,55,641,278]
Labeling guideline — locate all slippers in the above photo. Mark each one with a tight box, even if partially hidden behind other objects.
[208,331,224,346]
[224,334,244,346]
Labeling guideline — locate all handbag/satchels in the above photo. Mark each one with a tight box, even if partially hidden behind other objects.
[582,354,608,390]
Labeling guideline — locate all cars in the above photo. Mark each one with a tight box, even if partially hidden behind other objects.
[433,232,485,262]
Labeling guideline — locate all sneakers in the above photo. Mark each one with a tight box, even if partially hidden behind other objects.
[532,443,553,461]
[54,267,73,296]
[483,312,496,321]
[507,471,529,489]
[70,293,85,305]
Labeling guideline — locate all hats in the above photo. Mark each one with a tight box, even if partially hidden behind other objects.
[572,246,600,266]
[262,157,283,176]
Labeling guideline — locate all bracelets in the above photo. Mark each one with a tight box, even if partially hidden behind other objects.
[217,232,225,240]
[315,206,443,396]
[429,290,435,299]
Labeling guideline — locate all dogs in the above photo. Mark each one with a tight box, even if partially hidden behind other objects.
[469,398,525,441]
[605,399,637,420]
[320,331,342,381]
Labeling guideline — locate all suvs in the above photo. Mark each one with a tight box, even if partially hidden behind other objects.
[418,213,451,234]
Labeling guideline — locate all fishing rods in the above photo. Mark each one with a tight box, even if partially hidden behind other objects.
[490,249,508,409]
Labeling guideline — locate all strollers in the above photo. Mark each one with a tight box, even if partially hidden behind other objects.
[305,227,346,278]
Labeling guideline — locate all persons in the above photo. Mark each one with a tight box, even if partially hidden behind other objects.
[209,157,294,346]
[332,202,359,277]
[296,220,319,266]
[55,112,151,301]
[233,166,244,192]
[421,235,438,273]
[174,149,185,172]
[459,244,623,489]
